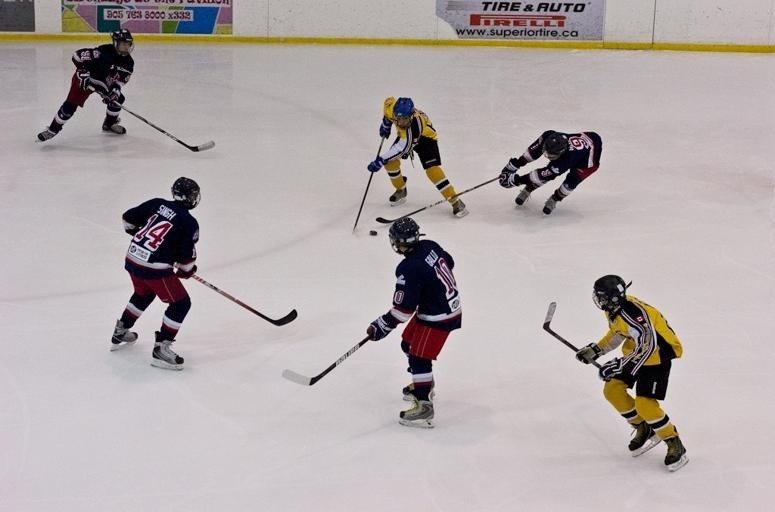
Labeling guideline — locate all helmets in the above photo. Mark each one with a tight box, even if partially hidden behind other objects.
[541,132,570,161]
[392,97,415,129]
[111,28,136,56]
[591,275,626,312]
[388,216,420,255]
[171,177,201,209]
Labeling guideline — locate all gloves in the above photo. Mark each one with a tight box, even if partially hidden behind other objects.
[77,69,89,91]
[575,342,604,365]
[379,116,392,139]
[598,356,625,382]
[174,263,198,279]
[366,309,405,342]
[367,156,387,173]
[102,83,121,104]
[502,157,520,174]
[498,172,520,188]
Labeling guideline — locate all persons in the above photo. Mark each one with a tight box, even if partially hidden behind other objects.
[367,215,462,420]
[499,130,602,214]
[38,28,134,141]
[367,98,465,215]
[576,274,687,465]
[112,176,201,365]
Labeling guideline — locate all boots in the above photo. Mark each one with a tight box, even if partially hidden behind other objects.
[152,330,185,365]
[389,175,407,202]
[514,186,534,205]
[37,124,62,142]
[450,194,466,216]
[542,190,564,215]
[111,319,138,344]
[664,425,687,466]
[627,420,655,451]
[100,122,128,134]
[402,375,435,396]
[400,391,434,421]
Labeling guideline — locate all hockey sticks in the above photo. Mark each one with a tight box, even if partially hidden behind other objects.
[353,136,384,236]
[112,99,215,152]
[281,336,373,387]
[375,176,503,223]
[543,301,602,369]
[191,273,297,326]
